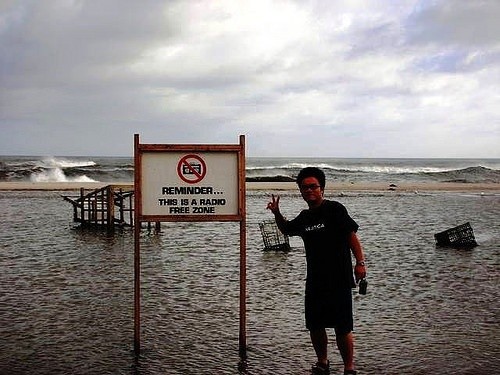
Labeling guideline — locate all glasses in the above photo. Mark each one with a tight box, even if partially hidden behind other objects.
[300,185,320,190]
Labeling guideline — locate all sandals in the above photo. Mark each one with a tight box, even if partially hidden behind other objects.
[311,359,330,375]
[343,370,358,375]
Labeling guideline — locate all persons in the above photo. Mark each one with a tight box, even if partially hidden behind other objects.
[266,165,366,375]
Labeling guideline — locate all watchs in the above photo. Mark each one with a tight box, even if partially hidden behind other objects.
[356,260,366,267]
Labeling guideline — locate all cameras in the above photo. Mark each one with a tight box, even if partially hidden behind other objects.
[359,279,367,295]
